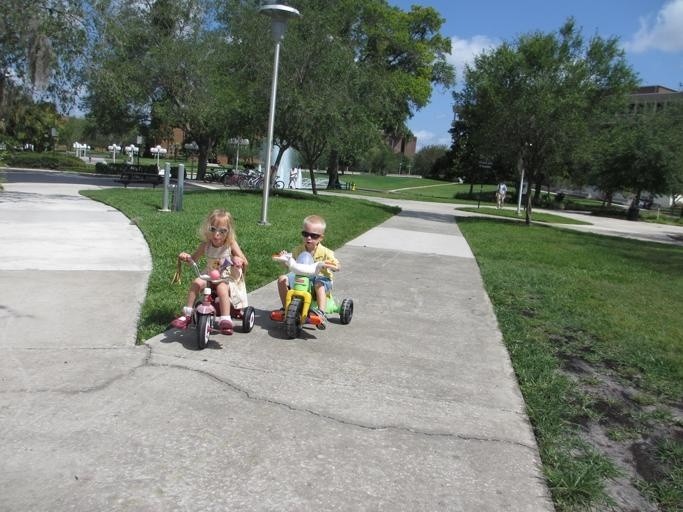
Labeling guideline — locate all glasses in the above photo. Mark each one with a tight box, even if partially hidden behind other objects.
[207,226,228,234]
[301,230,322,239]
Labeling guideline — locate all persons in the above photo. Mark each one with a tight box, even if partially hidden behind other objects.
[170,207,250,331]
[496,182,507,210]
[119,154,135,179]
[269,215,344,331]
[288,166,298,189]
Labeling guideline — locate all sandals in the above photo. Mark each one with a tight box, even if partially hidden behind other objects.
[219,319,233,336]
[170,315,192,331]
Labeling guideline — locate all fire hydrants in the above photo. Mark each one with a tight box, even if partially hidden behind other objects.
[351,184,356,191]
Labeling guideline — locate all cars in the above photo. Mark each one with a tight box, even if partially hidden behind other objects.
[558,186,592,199]
[629,196,661,210]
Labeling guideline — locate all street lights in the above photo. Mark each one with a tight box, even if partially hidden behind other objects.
[254,5,301,225]
[228,134,249,170]
[72,141,90,157]
[150,145,167,171]
[185,142,199,179]
[516,141,532,214]
[108,144,139,164]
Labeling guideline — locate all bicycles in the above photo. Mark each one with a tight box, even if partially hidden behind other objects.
[203,166,285,190]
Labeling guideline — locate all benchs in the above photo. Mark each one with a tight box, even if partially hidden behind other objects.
[112,172,161,188]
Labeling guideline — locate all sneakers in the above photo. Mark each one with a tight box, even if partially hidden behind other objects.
[308,306,326,331]
